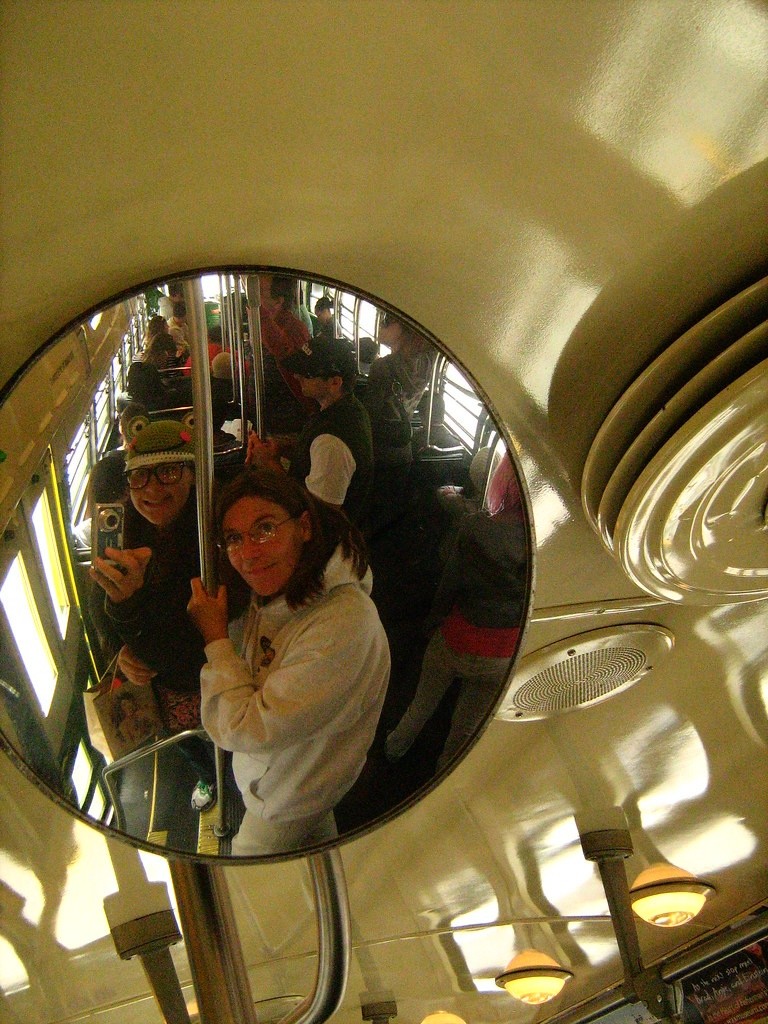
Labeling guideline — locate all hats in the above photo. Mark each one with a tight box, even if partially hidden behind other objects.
[279,335,358,376]
[121,421,196,472]
[315,297,334,310]
[468,445,502,492]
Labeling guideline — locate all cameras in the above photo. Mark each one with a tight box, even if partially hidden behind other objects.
[90,502,125,565]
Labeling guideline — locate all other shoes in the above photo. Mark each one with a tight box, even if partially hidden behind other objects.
[191,781,217,813]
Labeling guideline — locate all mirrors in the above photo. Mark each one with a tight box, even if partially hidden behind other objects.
[0,264,536,865]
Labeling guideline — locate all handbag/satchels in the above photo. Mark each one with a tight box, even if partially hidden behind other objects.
[82,645,164,762]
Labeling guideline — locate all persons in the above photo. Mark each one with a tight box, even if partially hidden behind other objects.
[109,694,161,748]
[374,441,533,774]
[79,274,435,856]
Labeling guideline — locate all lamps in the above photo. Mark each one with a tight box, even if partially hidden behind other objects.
[496,968,573,1005]
[630,882,717,927]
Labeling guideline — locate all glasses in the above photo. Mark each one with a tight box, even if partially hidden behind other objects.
[382,313,396,327]
[303,372,324,380]
[120,462,190,489]
[214,517,290,552]
